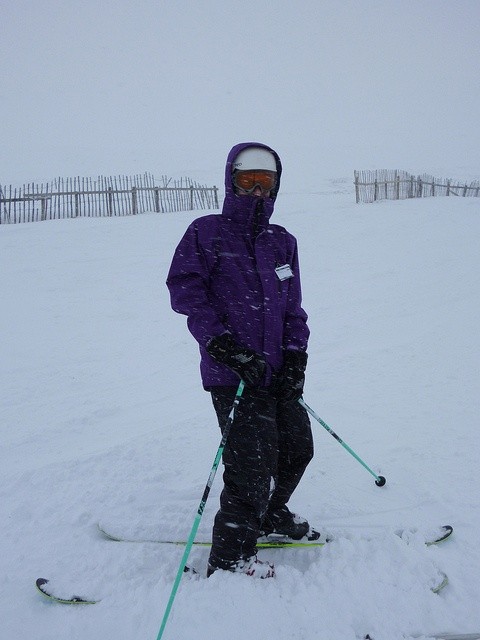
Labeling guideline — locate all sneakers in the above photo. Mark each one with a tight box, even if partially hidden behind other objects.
[261,507,309,540]
[207,557,274,578]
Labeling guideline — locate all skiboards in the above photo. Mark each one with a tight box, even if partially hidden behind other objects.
[36,517,452,605]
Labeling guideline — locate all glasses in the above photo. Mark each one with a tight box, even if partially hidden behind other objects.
[233,171,278,194]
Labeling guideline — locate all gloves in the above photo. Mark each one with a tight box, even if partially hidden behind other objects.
[279,348,308,407]
[207,331,267,388]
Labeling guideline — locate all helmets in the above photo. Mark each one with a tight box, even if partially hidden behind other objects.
[232,149,276,171]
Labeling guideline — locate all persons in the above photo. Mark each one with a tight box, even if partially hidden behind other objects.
[165,142,314,578]
